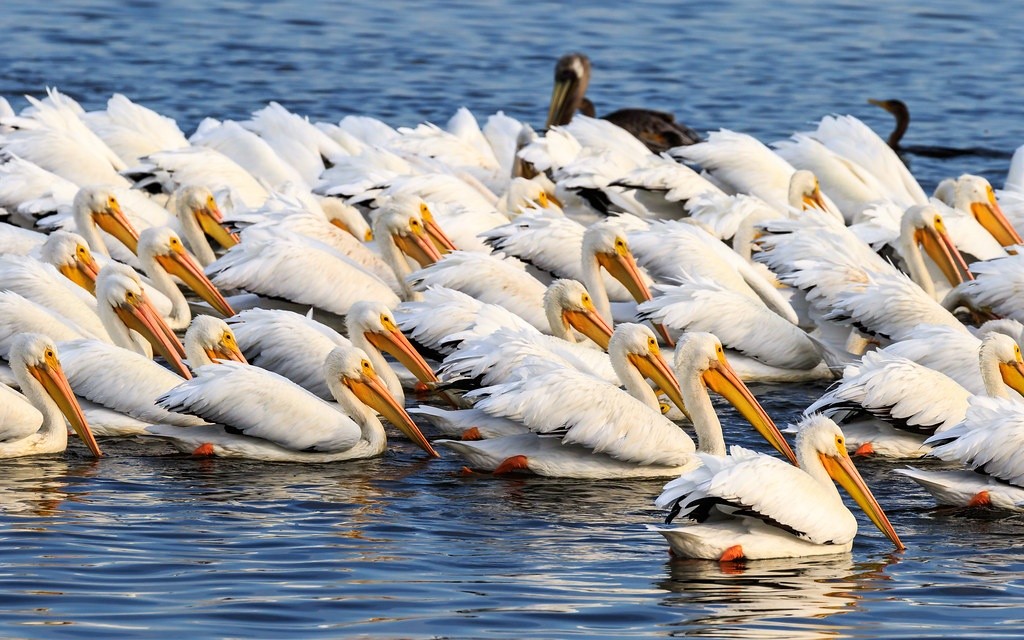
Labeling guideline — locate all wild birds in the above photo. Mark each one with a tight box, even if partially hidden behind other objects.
[0,84,1024,511]
[867,98,1015,183]
[547,53,701,156]
[644,414,904,563]
